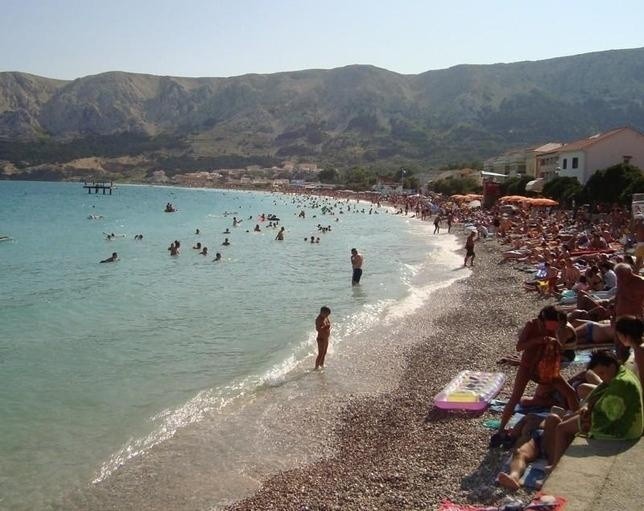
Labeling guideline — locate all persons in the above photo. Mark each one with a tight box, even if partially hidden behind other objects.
[350,248,364,287]
[331,191,380,221]
[494,264,643,491]
[463,202,643,296]
[315,306,330,369]
[100,198,331,265]
[381,189,468,234]
